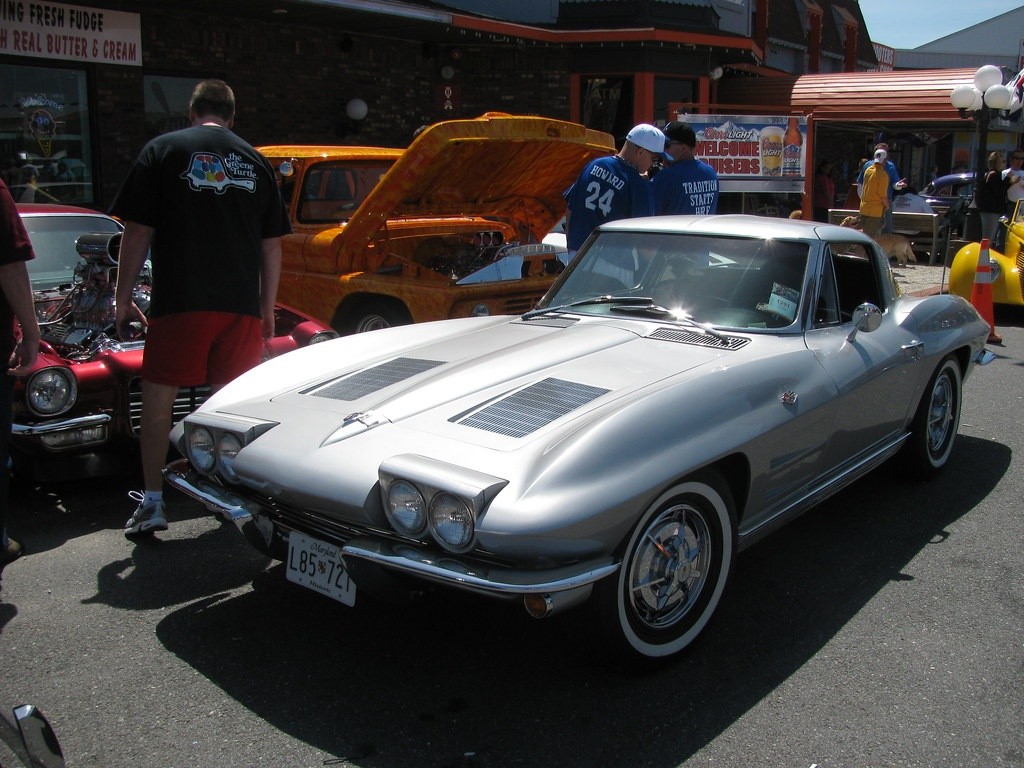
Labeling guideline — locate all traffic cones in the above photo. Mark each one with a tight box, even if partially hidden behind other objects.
[971,237,1002,345]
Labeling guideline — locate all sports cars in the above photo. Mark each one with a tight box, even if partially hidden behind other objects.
[160,214,993,673]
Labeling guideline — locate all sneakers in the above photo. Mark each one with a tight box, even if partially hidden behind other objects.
[123,490,168,535]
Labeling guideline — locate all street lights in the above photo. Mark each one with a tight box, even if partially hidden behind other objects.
[952,64,1011,244]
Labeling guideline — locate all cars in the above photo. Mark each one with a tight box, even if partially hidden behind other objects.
[8,202,343,468]
[947,198,1024,306]
[915,172,988,220]
[251,111,619,336]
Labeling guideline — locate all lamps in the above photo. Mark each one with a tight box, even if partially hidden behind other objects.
[333,98,368,139]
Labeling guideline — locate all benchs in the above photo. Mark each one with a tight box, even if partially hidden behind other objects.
[827,207,950,267]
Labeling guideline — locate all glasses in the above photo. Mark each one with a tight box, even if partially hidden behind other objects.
[1013,157,1024,161]
[664,140,681,146]
[643,152,660,164]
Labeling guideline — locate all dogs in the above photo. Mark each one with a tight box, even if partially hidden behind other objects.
[839,215,916,268]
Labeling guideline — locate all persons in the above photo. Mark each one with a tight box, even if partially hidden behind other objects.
[815,161,835,224]
[563,124,674,290]
[997,149,1024,251]
[977,151,1021,247]
[650,121,719,280]
[108,79,295,533]
[839,143,908,239]
[0,176,40,556]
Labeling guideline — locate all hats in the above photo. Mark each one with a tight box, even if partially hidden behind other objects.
[662,122,696,146]
[874,149,887,164]
[626,123,674,161]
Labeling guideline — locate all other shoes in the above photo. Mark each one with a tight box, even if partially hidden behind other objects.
[0,535,23,564]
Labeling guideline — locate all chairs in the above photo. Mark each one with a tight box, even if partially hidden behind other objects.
[746,254,808,321]
